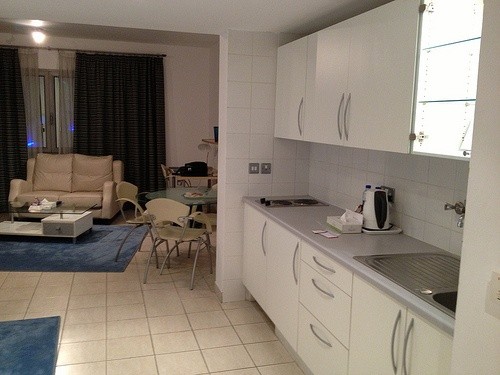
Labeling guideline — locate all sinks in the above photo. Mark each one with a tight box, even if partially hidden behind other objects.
[431,291,458,314]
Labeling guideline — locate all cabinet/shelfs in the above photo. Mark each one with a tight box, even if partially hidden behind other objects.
[241,202,451,375]
[272,0,423,155]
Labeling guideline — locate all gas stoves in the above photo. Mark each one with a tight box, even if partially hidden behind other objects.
[254,197,330,209]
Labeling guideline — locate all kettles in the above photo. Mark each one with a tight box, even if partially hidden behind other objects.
[362,189,390,231]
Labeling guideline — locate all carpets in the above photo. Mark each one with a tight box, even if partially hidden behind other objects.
[0,314,61,375]
[0,221,152,273]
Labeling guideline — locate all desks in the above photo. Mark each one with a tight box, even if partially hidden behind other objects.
[146,186,217,268]
[173,175,218,189]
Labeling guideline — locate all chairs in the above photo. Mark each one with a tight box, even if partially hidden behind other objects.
[114,181,179,269]
[159,163,175,187]
[186,183,217,258]
[143,196,212,291]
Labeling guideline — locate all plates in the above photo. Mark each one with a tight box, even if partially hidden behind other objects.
[362,225,402,234]
[184,192,207,198]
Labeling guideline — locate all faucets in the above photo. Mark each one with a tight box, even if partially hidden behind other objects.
[456,214,464,229]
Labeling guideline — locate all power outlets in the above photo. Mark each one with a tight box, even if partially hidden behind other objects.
[260,163,271,174]
[248,162,259,174]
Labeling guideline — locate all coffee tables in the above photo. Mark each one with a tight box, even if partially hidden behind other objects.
[0,202,98,245]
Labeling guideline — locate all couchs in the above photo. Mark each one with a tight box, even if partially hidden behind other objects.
[8,153,125,225]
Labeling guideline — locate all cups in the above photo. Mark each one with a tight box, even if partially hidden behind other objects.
[214,127,218,142]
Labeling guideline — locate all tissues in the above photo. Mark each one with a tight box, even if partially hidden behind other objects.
[326,208,364,235]
[40,198,56,207]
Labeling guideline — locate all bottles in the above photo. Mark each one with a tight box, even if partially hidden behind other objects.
[362,184,372,210]
[375,187,381,189]
